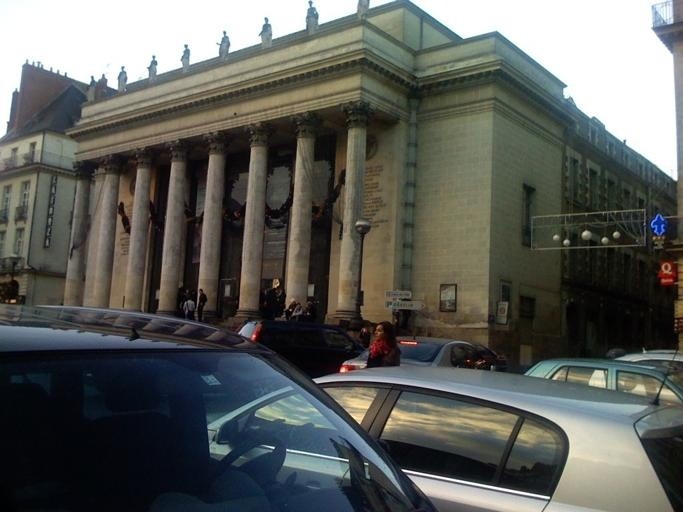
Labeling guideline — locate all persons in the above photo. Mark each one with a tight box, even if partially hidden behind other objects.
[81,0,321,105]
[174,284,403,372]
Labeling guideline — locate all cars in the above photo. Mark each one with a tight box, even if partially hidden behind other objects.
[0,302,435,510]
[519,358,683,410]
[339,335,509,375]
[206,366,683,512]
[587,347,683,395]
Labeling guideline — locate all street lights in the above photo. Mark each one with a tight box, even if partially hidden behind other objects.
[7,254,20,303]
[354,218,371,318]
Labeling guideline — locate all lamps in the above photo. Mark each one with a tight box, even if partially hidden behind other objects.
[544,214,626,247]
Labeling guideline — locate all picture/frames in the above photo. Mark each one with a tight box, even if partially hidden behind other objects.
[439,283,457,312]
[494,300,509,325]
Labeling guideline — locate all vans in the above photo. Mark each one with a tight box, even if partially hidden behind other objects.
[235,318,365,380]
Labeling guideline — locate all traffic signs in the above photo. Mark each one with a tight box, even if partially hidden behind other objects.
[384,289,413,300]
[383,301,424,311]
